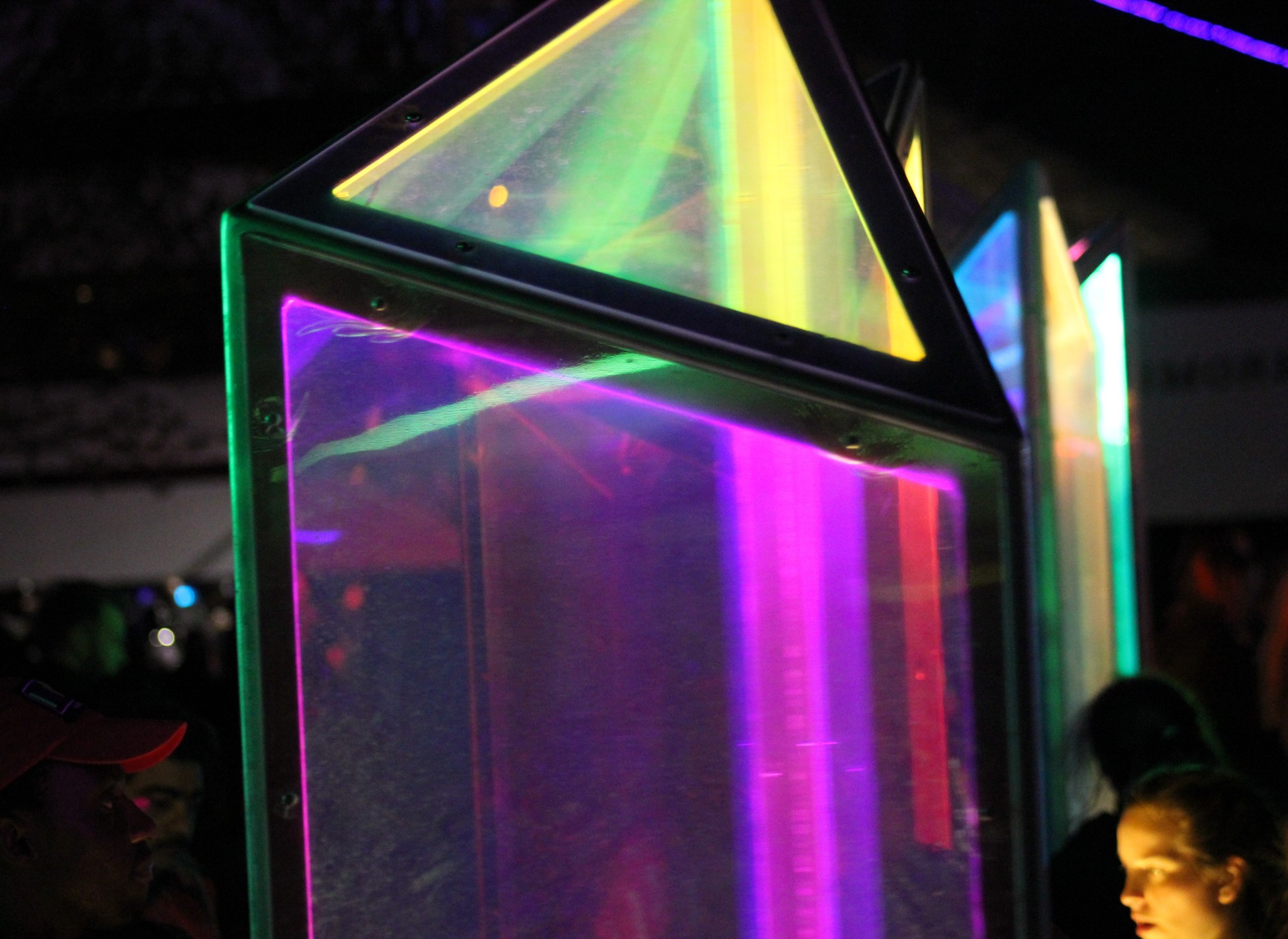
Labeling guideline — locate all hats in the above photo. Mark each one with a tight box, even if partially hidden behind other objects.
[1,705,187,786]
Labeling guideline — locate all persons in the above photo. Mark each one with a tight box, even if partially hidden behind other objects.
[1048,677,1218,938]
[4,704,246,939]
[1115,764,1287,939]
